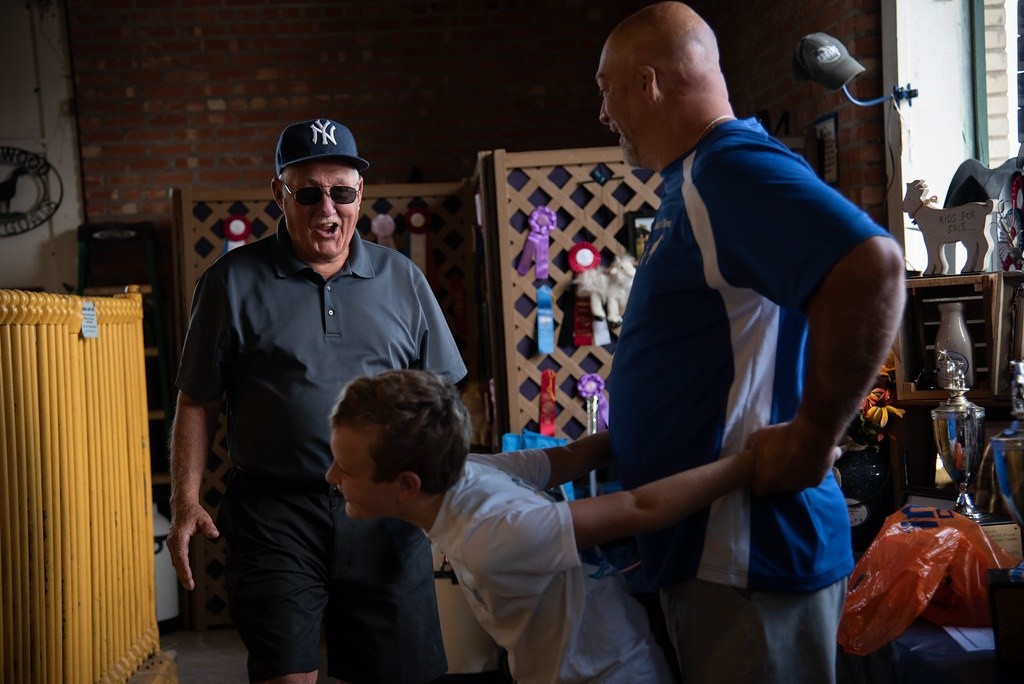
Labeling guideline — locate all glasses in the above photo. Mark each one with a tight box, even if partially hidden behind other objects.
[281,181,360,206]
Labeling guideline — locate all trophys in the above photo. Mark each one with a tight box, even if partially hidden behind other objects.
[931,349,992,525]
[989,361,1024,581]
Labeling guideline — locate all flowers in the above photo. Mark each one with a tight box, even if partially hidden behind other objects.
[856,365,905,443]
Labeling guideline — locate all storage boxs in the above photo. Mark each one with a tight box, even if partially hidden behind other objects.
[893,272,1017,400]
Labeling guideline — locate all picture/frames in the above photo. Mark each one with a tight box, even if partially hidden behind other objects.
[625,211,656,258]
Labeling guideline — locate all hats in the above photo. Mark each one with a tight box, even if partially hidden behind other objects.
[792,32,866,91]
[275,119,370,178]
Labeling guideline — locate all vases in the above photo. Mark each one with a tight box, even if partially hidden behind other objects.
[934,302,979,389]
[841,442,884,502]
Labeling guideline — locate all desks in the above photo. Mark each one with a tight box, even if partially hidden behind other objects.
[867,617,1024,684]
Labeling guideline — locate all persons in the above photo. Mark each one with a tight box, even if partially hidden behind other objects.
[326,370,844,684]
[168,119,468,684]
[596,1,907,684]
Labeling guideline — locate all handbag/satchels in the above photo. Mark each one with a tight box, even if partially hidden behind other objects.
[836,503,1020,657]
